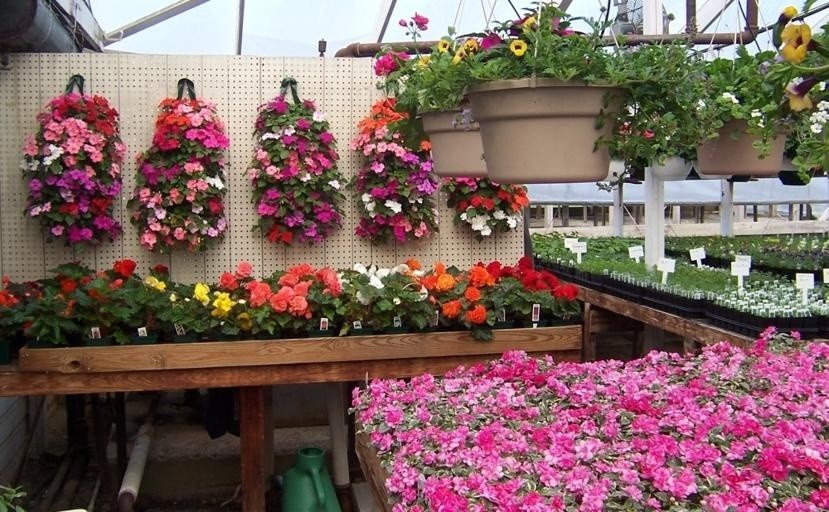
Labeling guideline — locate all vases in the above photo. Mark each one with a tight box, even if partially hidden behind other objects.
[726,174,751,183]
[625,159,648,183]
[600,154,630,183]
[650,148,693,181]
[780,154,813,173]
[467,77,628,186]
[777,168,816,189]
[687,102,788,174]
[686,155,734,182]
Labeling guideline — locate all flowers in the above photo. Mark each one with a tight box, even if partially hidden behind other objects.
[431,172,530,242]
[344,323,829,511]
[15,90,130,250]
[0,256,584,342]
[348,96,439,248]
[589,6,829,162]
[368,1,634,129]
[128,96,233,252]
[239,87,348,249]
